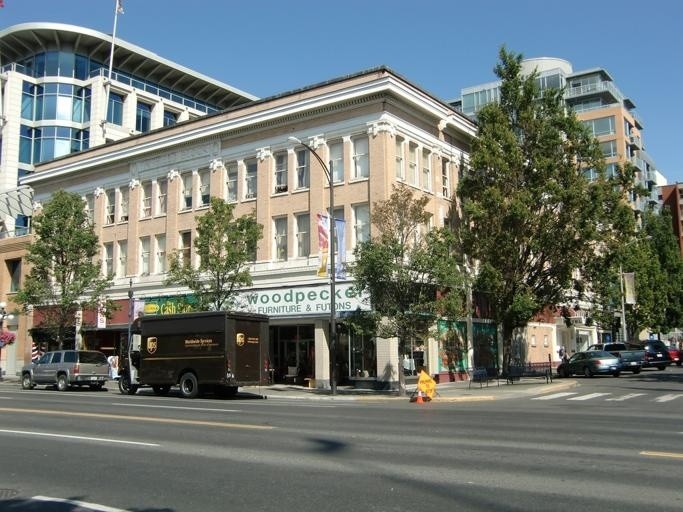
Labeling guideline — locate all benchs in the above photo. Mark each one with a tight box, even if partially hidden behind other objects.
[466,366,500,389]
[504,366,524,386]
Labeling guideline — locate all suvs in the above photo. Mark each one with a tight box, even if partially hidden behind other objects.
[20,349,111,391]
[635,340,671,369]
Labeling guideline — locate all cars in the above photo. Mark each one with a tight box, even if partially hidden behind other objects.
[663,346,682,366]
[555,348,624,379]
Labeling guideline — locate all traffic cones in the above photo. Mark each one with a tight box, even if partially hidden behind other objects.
[415,386,423,404]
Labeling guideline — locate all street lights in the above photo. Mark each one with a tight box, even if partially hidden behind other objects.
[124,277,132,346]
[616,235,654,341]
[284,135,338,394]
[0,301,14,382]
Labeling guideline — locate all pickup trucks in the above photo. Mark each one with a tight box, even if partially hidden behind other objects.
[585,341,647,375]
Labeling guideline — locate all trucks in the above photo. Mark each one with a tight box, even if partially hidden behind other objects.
[118,310,274,397]
